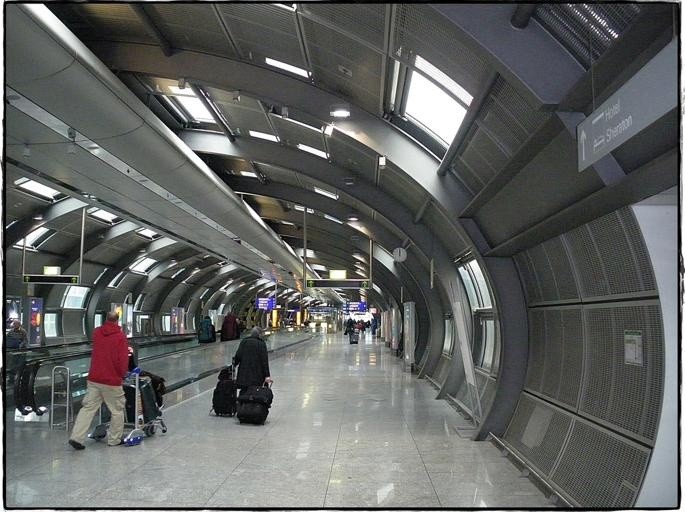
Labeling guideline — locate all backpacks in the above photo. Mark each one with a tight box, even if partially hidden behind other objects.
[223,316,237,339]
[198,318,212,341]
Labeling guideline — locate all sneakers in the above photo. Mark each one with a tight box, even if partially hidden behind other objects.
[108,438,126,446]
[68,439,85,449]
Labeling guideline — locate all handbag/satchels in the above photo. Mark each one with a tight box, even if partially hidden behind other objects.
[139,369,166,407]
[218,365,235,380]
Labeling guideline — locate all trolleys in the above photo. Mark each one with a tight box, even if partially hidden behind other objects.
[87,367,167,447]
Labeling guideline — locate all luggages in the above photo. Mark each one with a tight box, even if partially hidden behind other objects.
[122,373,162,423]
[213,357,237,417]
[237,379,273,425]
[350,335,358,344]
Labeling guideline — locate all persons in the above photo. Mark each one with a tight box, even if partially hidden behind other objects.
[343,317,371,336]
[231,326,270,390]
[6,320,27,385]
[268,319,272,327]
[220,312,240,342]
[68,311,129,450]
[197,316,216,346]
[276,320,281,327]
